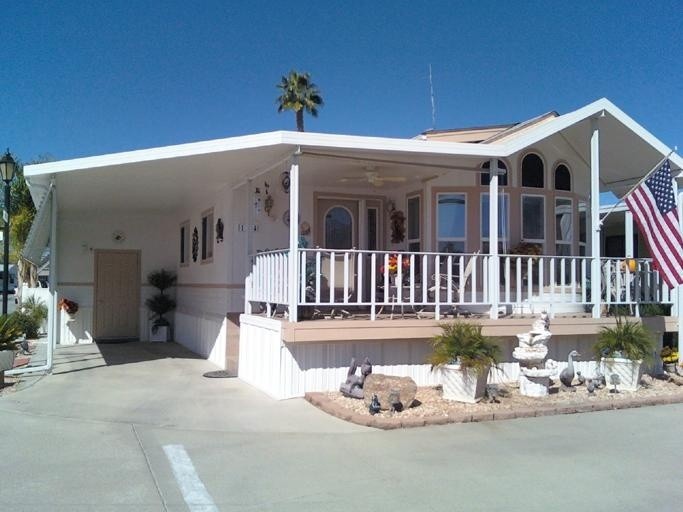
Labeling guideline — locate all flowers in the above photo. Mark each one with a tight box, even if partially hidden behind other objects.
[377,255,411,274]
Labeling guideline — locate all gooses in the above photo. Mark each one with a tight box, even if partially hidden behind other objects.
[559,349,582,387]
[587,378,595,394]
[577,371,587,384]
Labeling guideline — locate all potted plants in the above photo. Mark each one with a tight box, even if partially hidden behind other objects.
[144,267,179,343]
[0,310,25,373]
[424,321,510,405]
[589,310,661,392]
[58,298,80,319]
[18,294,48,355]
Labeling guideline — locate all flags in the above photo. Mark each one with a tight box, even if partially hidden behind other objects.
[623,160,683,290]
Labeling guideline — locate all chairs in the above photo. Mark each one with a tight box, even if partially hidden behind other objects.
[313,245,360,320]
[428,249,481,304]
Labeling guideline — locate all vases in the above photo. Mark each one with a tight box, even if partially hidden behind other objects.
[390,274,398,285]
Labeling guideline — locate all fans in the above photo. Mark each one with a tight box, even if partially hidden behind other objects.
[336,163,409,190]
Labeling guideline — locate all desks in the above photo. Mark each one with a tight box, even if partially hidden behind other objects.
[376,285,421,320]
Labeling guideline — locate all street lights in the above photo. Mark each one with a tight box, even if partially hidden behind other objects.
[0,144,20,318]
